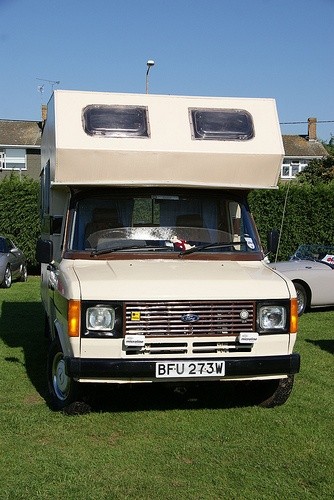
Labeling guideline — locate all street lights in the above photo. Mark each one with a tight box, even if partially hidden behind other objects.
[144,59,155,94]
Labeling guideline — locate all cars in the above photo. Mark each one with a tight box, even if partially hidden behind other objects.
[0,235,28,289]
[267,242,334,317]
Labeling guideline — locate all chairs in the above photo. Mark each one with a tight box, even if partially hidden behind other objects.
[176,214,211,241]
[84,205,126,239]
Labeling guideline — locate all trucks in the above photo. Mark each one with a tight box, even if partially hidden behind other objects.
[33,88,301,415]
